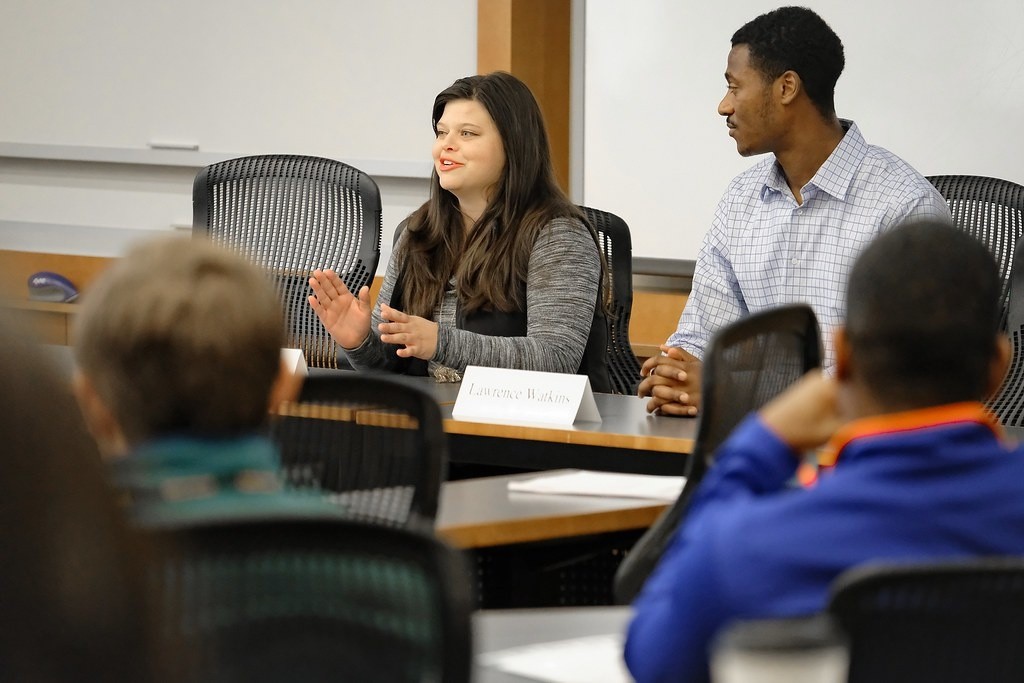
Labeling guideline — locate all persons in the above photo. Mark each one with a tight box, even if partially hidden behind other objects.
[306,70,609,395]
[637,7,954,439]
[1,229,457,683]
[623,222,1024,683]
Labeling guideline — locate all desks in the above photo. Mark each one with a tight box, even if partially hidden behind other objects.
[41,343,461,506]
[472,603,636,683]
[354,391,699,453]
[333,468,689,555]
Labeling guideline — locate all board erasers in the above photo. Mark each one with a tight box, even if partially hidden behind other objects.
[151,139,197,152]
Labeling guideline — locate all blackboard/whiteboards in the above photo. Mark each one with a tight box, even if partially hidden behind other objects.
[0,0,483,180]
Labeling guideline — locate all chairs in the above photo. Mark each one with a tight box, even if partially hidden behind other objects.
[828,560,1024,683]
[615,303,825,606]
[271,372,447,529]
[190,151,382,371]
[925,175,1024,429]
[580,204,643,397]
[145,513,478,683]
